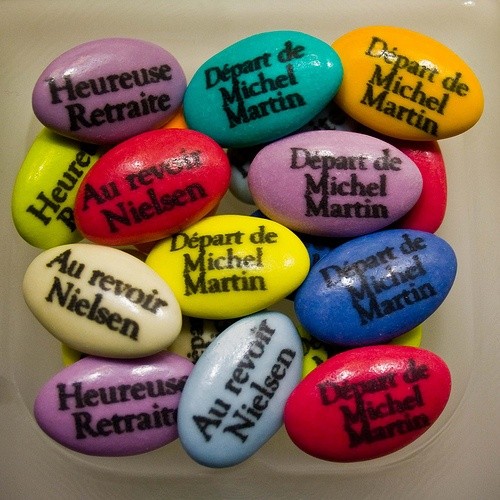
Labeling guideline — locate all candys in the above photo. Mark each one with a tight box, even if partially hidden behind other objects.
[12,25,484,469]
